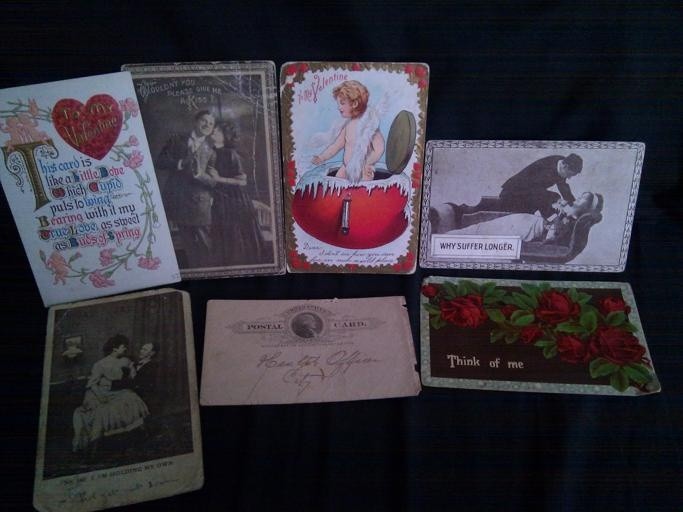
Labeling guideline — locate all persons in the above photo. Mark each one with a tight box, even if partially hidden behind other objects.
[311,79,385,182]
[204,118,267,266]
[118,342,176,451]
[74,334,149,473]
[458,149,605,247]
[154,110,218,228]
[293,314,320,339]
